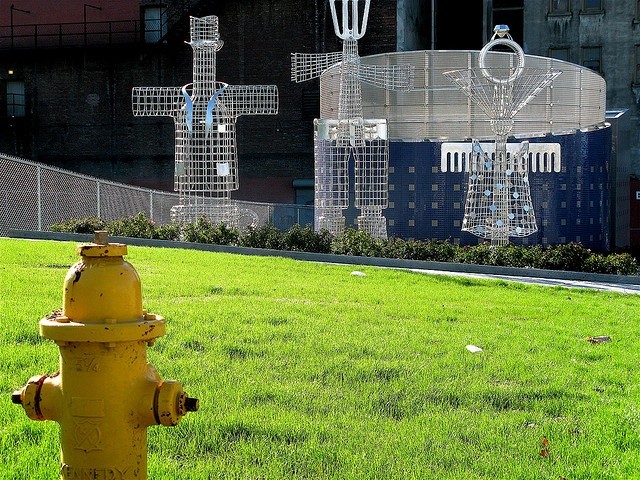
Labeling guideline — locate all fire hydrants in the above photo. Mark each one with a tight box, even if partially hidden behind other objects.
[11,231,200,480]
